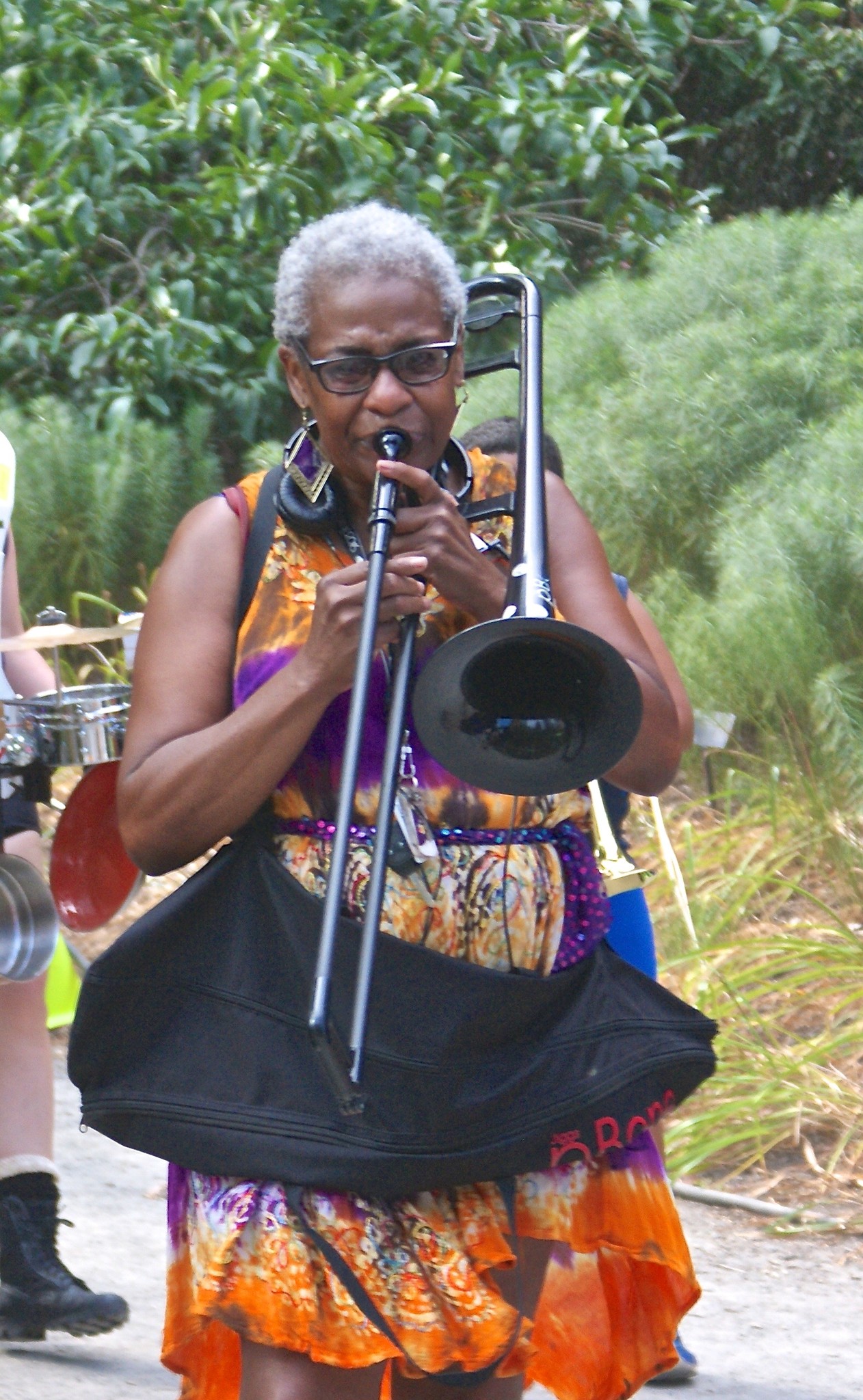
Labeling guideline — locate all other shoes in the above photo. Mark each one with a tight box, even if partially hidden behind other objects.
[649,1338,698,1383]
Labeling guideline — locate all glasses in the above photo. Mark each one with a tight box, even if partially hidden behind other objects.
[294,318,460,395]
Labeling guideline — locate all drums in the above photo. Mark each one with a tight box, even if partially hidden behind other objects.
[117,611,146,671]
[16,684,135,766]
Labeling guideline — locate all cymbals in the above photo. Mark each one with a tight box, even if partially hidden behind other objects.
[0,623,136,654]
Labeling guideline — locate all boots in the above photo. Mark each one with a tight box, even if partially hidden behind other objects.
[1,1170,129,1343]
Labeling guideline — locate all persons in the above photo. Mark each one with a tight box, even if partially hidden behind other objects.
[119,205,683,1400]
[0,431,700,1386]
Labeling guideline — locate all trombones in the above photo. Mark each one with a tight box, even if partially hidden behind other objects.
[304,271,638,1121]
